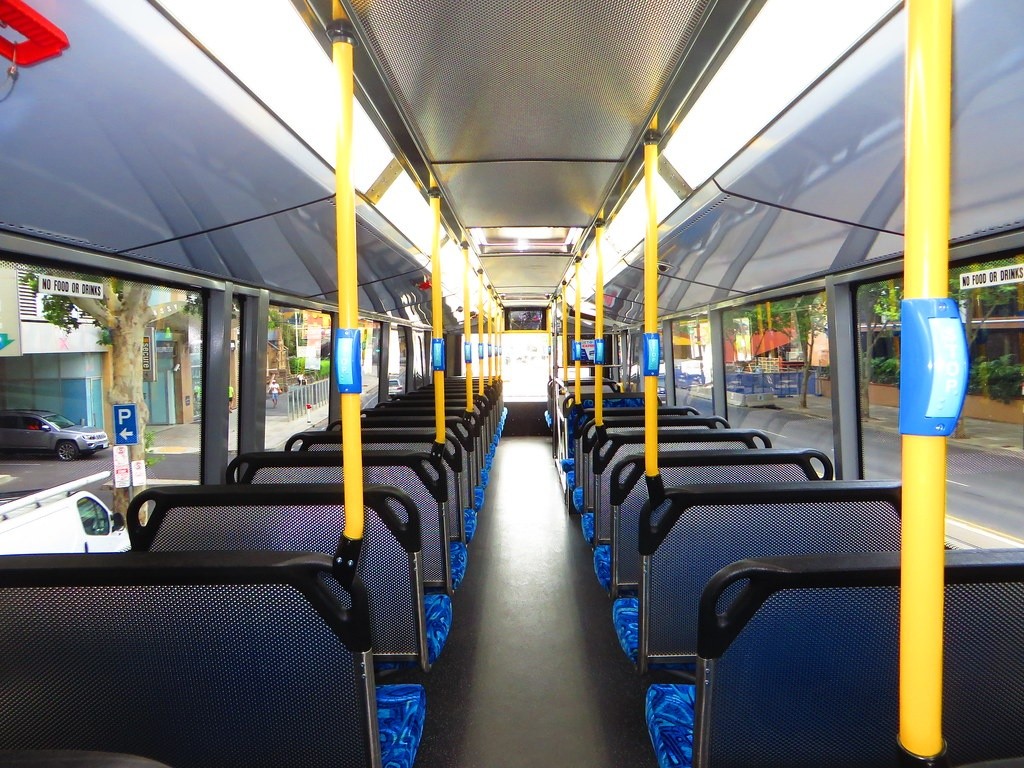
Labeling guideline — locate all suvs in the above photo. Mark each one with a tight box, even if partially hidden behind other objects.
[0,409,110,461]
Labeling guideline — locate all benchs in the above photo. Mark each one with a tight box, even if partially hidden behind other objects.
[0,376,1024,767]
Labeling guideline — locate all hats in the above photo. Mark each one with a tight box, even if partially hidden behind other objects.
[272,380,276,383]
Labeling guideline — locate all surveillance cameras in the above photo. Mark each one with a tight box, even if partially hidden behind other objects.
[545,293,551,299]
[501,295,506,300]
[458,307,463,311]
[656,262,670,272]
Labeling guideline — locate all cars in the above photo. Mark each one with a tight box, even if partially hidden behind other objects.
[388,378,404,399]
[655,373,705,400]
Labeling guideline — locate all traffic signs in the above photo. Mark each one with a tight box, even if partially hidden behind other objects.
[111,405,140,447]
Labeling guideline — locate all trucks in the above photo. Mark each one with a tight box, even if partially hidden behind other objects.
[0,471,132,554]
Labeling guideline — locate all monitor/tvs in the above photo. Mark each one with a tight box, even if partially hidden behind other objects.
[566,334,613,366]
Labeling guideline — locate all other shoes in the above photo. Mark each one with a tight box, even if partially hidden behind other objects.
[230,411,232,413]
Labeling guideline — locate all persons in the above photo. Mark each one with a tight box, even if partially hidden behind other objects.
[269,380,280,408]
[229,386,233,413]
[297,372,307,385]
